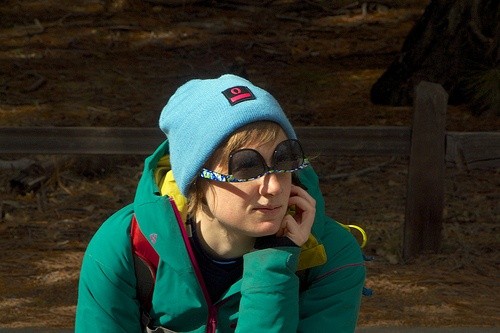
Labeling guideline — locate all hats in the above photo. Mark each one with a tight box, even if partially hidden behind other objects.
[159,73,297,196]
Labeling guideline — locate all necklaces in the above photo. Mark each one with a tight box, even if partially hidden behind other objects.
[210,259,237,265]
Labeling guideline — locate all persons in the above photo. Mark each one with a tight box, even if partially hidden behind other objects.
[73,73,374,333]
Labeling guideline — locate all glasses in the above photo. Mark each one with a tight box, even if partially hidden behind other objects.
[197,137,310,183]
